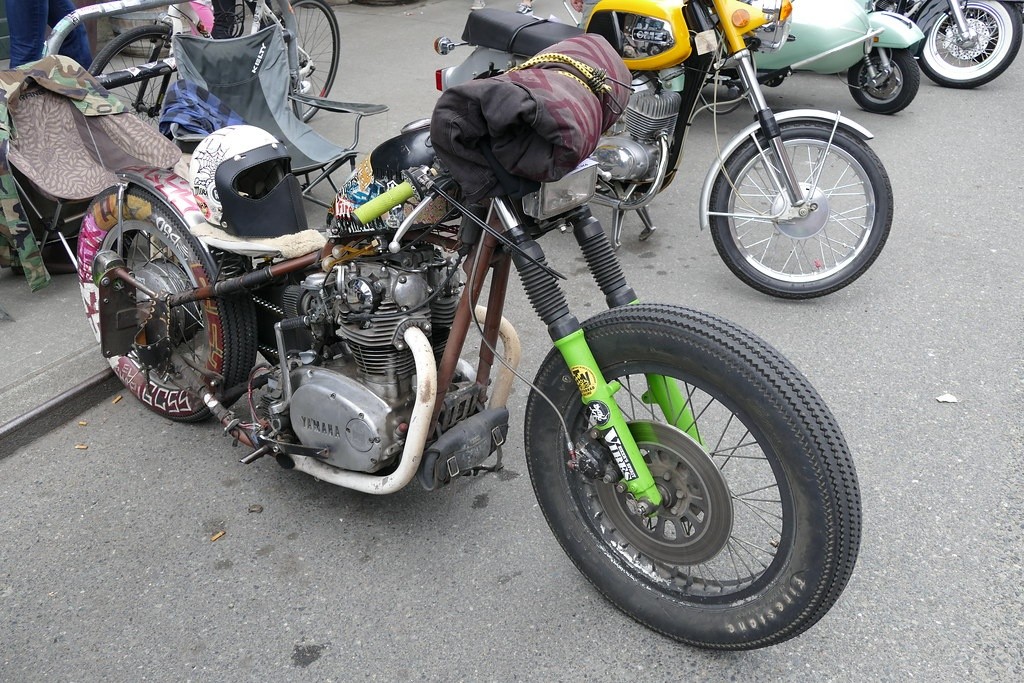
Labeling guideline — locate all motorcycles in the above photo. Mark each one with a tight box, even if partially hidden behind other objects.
[78,0,1023,650]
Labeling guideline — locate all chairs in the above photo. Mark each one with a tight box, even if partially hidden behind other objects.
[0,55,181,273]
[169,26,390,208]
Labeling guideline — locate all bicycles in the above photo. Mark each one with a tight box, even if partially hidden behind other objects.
[85,0,339,142]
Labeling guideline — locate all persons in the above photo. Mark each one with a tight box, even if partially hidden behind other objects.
[211,0,311,94]
[5,0,95,71]
[189,0,216,36]
[571,0,601,28]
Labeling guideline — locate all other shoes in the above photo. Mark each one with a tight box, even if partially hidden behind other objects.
[299,80,311,95]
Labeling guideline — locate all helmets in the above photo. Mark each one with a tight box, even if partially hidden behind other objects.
[186,120,315,236]
